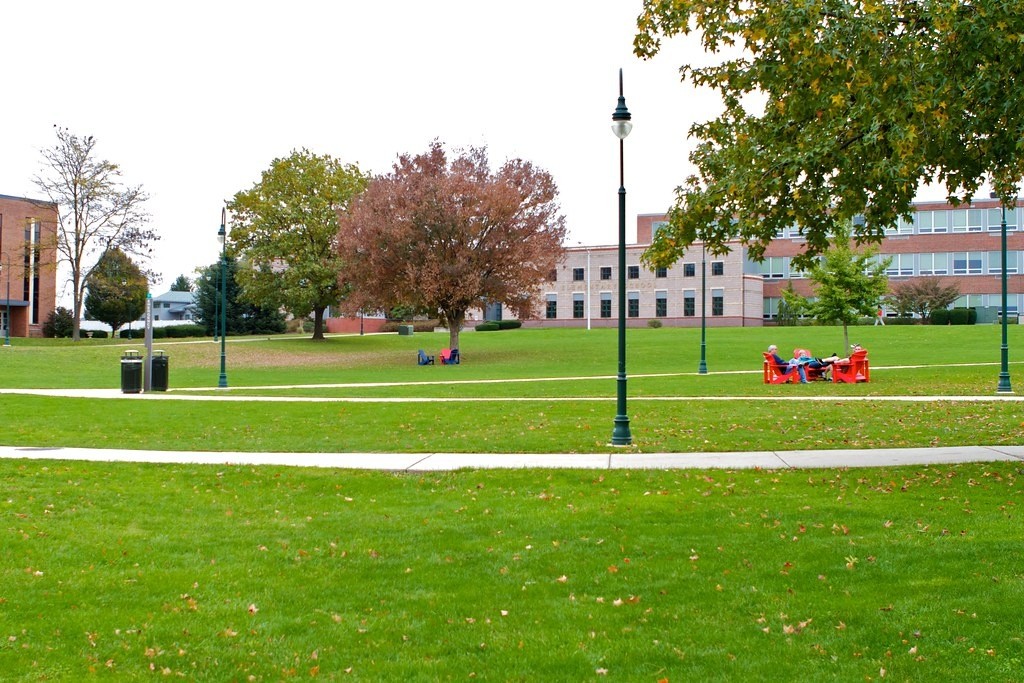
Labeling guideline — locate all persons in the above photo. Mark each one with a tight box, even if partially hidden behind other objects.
[768,344,811,384]
[797,349,837,368]
[815,344,861,381]
[874,306,885,325]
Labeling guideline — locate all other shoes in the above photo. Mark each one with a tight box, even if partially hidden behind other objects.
[799,362,809,369]
[829,377,832,383]
[815,358,823,365]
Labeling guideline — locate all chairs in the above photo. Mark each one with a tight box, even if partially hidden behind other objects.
[794,347,827,381]
[832,349,870,384]
[763,352,797,385]
[442,349,460,365]
[417,349,434,365]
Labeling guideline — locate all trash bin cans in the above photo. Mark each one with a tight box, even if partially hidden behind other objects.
[151,349,171,391]
[120,349,144,394]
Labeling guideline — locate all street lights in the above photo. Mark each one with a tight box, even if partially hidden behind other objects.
[0,250,11,345]
[613,66,632,447]
[217,207,228,389]
